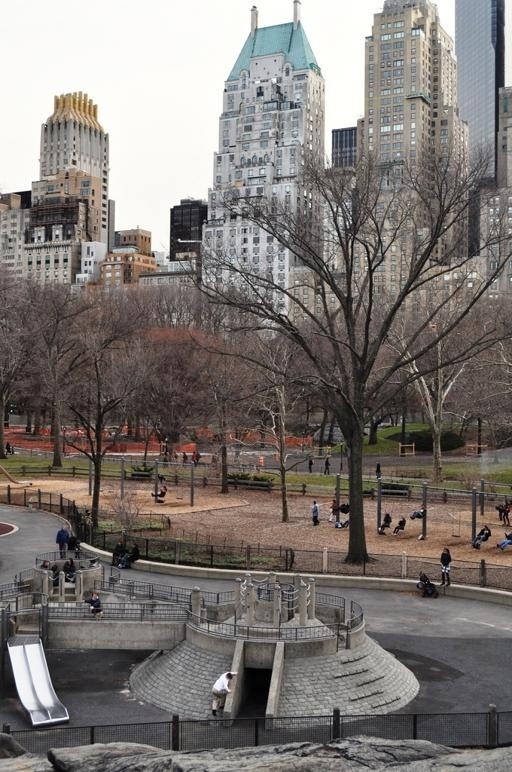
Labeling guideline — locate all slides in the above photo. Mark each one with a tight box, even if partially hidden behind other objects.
[7,639,69,726]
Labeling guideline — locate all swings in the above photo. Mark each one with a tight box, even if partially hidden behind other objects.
[175,464,184,499]
[504,496,512,545]
[383,484,424,530]
[159,464,173,497]
[477,494,497,541]
[129,461,138,495]
[451,490,462,537]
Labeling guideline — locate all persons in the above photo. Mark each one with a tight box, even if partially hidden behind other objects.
[410,503,427,520]
[210,672,239,716]
[391,517,406,536]
[110,540,140,569]
[308,455,313,473]
[42,525,98,587]
[6,441,11,455]
[164,449,201,466]
[213,451,218,464]
[440,546,452,586]
[157,474,167,484]
[471,500,512,550]
[82,591,103,622]
[313,499,350,529]
[379,513,392,534]
[151,485,168,497]
[324,458,330,475]
